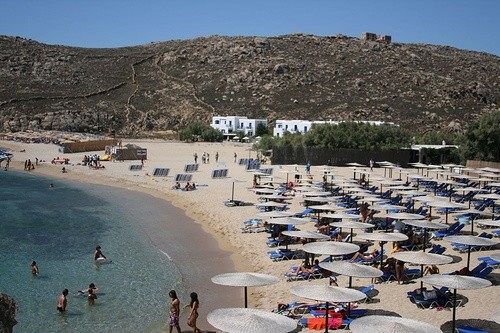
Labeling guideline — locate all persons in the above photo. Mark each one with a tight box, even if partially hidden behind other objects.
[94,245,106,260]
[289,259,319,275]
[350,248,379,262]
[391,241,405,285]
[424,264,439,276]
[78,283,97,301]
[306,161,311,175]
[234,152,237,162]
[172,181,180,190]
[57,289,68,312]
[184,182,192,190]
[360,202,369,223]
[169,289,180,333]
[6,154,104,173]
[194,151,218,163]
[184,292,201,333]
[31,260,39,275]
[370,159,374,172]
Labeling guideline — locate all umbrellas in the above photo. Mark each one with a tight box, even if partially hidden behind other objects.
[210,272,280,307]
[246,157,500,333]
[220,177,247,200]
[206,307,297,333]
[233,135,261,140]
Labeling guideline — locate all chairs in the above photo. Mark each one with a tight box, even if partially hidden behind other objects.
[241,174,500,333]
